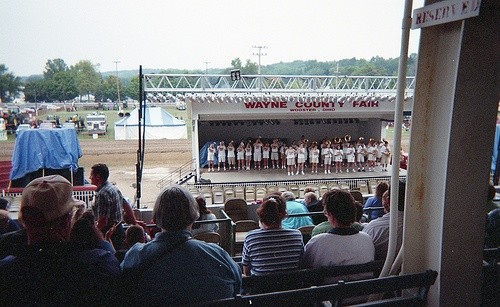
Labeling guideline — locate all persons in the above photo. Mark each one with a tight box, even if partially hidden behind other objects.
[0,114,500,307]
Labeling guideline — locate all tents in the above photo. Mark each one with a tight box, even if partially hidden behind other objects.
[114,107,187,141]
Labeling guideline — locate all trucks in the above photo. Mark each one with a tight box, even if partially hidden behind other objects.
[87,111,108,136]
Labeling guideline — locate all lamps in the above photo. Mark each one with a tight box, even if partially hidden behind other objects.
[231,70,241,80]
[209,120,280,126]
[293,118,360,125]
[146,92,413,110]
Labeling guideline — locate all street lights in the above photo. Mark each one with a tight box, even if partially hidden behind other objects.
[202,61,212,75]
[113,61,121,112]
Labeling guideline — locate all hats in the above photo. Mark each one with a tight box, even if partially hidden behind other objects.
[20,174,85,222]
[281,191,295,198]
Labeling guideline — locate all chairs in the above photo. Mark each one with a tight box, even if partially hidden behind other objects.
[298,225,314,245]
[194,199,259,253]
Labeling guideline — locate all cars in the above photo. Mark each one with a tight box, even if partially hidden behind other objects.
[60,123,79,134]
[37,122,56,129]
[15,124,31,137]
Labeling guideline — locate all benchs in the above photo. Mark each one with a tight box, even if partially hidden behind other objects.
[189,260,438,307]
